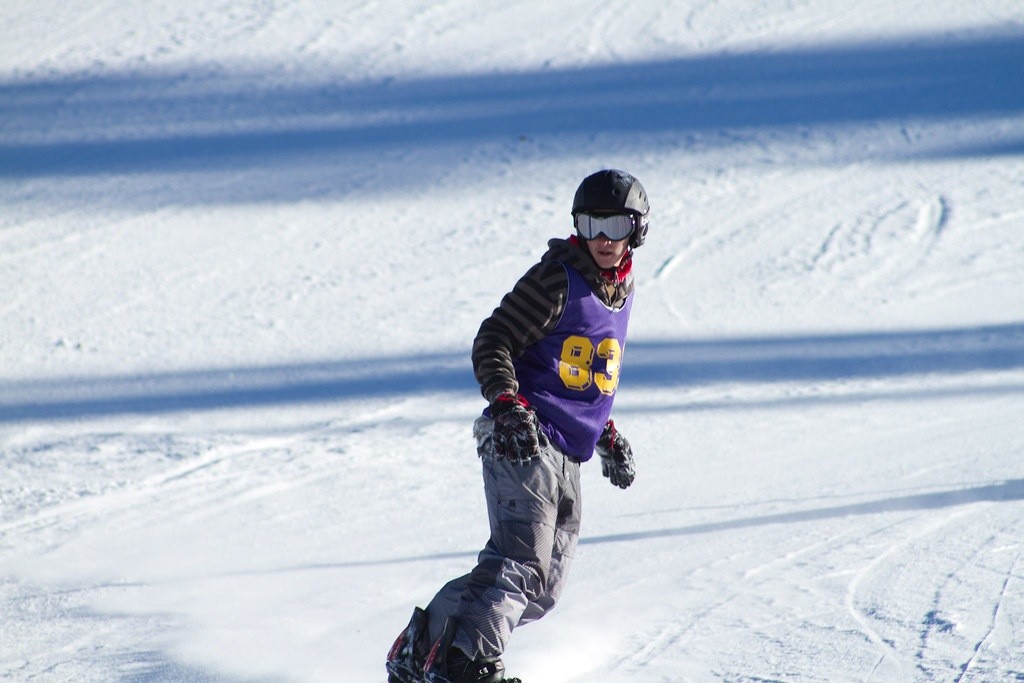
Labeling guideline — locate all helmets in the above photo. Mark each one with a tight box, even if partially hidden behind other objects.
[571,169,655,248]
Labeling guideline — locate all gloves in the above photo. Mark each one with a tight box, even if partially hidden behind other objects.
[490,391,548,467]
[596,419,635,489]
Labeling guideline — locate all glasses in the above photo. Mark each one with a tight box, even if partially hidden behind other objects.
[575,208,650,240]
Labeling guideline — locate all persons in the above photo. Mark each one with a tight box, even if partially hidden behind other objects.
[383,168,654,683]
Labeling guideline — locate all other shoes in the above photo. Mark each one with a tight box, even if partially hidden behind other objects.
[386,606,434,683]
[423,615,523,683]
